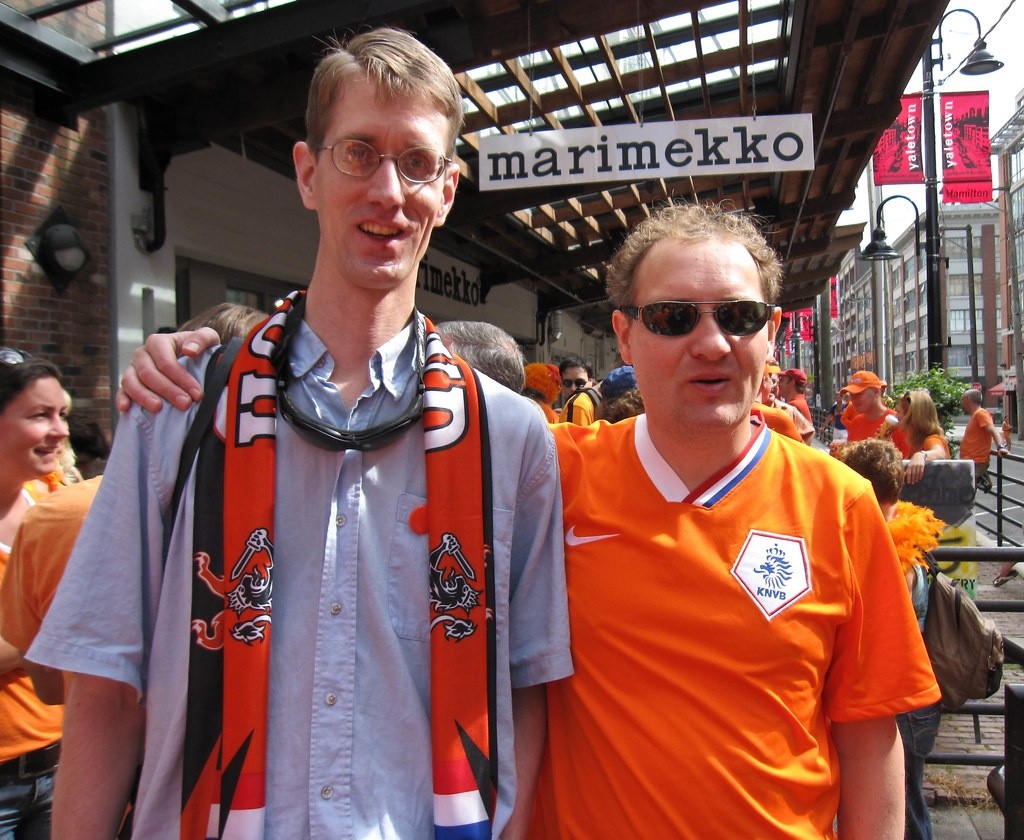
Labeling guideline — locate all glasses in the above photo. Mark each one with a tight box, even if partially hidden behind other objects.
[0,346,33,365]
[562,378,586,388]
[315,141,452,184]
[904,391,911,404]
[619,299,776,337]
[277,379,425,451]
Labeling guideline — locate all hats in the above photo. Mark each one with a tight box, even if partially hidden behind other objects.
[778,369,807,387]
[602,365,636,398]
[841,370,882,394]
[524,363,561,405]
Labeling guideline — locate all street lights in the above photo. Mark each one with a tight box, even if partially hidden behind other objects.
[859,193,948,414]
[923,7,1006,375]
[790,315,820,407]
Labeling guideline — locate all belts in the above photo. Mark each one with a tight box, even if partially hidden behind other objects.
[0,742,61,781]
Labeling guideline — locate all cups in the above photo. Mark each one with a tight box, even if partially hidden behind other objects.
[875,415,900,441]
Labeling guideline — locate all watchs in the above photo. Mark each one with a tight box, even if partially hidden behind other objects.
[997,444,1005,450]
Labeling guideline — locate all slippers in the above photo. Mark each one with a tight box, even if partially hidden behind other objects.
[993,570,1017,587]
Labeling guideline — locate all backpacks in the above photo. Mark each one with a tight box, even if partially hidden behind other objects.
[919,548,1004,709]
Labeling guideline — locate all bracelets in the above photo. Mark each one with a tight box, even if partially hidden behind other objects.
[914,450,928,462]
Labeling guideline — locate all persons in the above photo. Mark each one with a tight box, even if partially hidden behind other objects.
[0,300,278,839]
[23,25,574,840]
[114,202,941,839]
[749,360,1023,840]
[433,320,645,428]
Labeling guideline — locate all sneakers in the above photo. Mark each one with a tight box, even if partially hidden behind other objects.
[977,471,992,494]
